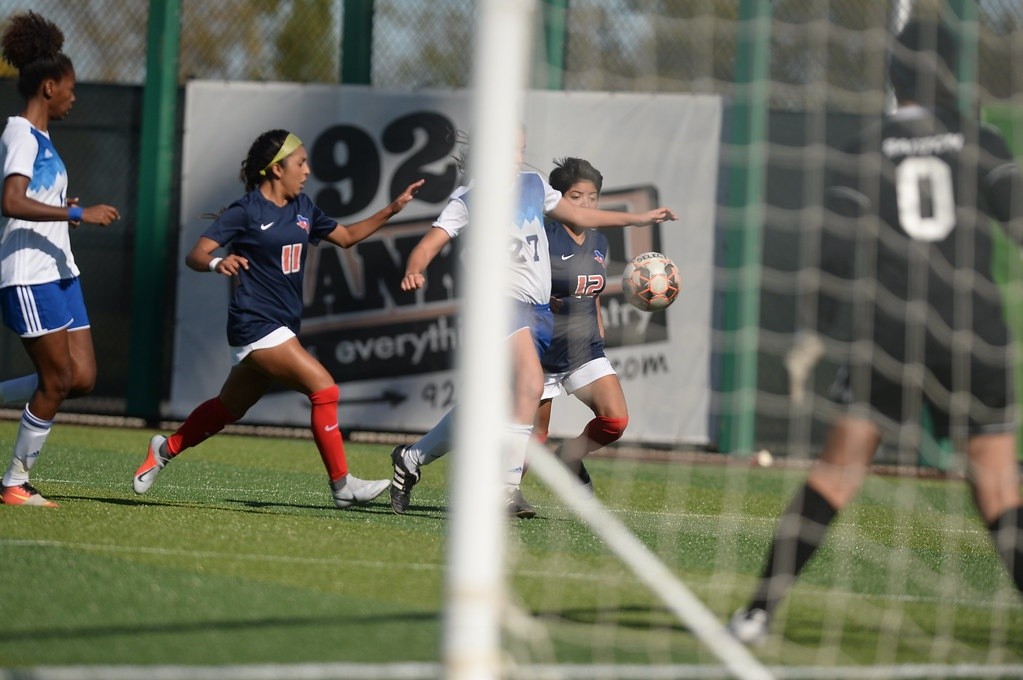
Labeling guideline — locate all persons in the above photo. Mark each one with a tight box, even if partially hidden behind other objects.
[0,9,122,508]
[132,128,426,508]
[729,14,1023,642]
[393,124,676,517]
[522,153,628,515]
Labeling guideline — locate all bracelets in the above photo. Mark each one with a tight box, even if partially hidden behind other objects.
[209,256,223,273]
[69,206,81,220]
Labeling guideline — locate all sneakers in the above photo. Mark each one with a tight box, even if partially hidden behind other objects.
[554,446,594,495]
[504,487,536,518]
[389,444,421,515]
[328,473,391,509]
[133,434,171,495]
[0,479,58,507]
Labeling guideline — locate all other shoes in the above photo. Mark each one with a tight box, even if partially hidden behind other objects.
[730,606,769,643]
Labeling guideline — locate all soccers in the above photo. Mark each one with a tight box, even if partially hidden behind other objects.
[621,250,681,313]
